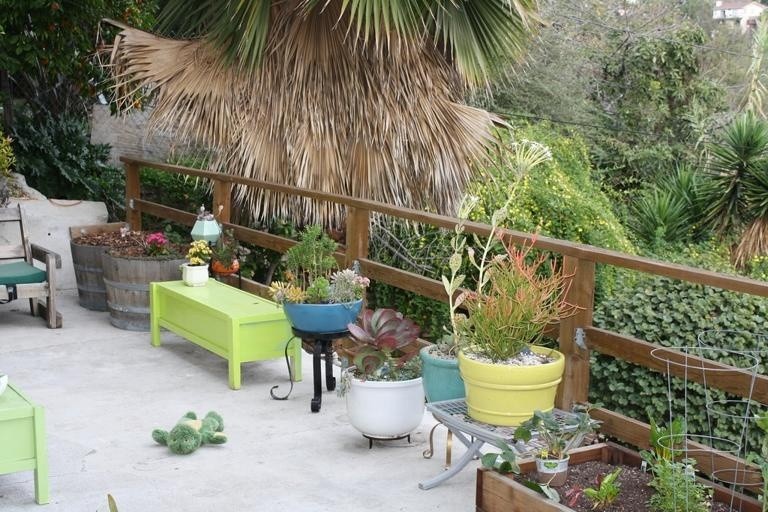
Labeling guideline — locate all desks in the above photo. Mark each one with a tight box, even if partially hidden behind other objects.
[149,278,303,391]
[270,326,350,412]
[418,397,596,491]
[0,378,50,504]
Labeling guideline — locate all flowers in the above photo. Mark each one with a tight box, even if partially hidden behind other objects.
[435,137,554,353]
[141,232,170,256]
[189,241,212,266]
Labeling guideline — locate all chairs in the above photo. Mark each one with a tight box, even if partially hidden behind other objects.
[0,201,63,329]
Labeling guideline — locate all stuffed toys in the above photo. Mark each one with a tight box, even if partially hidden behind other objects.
[152,411,228,455]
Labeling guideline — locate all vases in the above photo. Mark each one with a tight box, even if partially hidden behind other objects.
[101,245,190,331]
[182,262,210,286]
[70,231,128,311]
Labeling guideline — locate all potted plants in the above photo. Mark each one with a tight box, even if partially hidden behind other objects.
[332,308,427,438]
[475,440,767,512]
[419,194,509,403]
[453,223,588,426]
[212,225,240,276]
[268,223,370,333]
[481,401,605,487]
[191,204,222,247]
